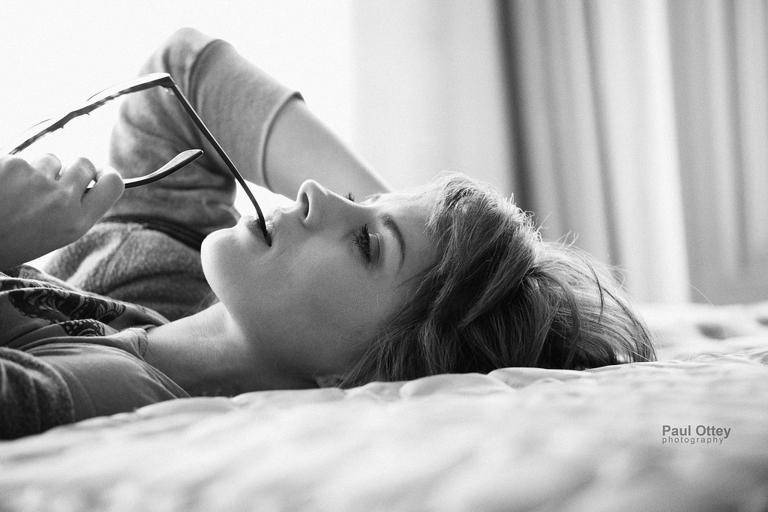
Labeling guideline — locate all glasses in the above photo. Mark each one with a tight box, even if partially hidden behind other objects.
[7,73,272,250]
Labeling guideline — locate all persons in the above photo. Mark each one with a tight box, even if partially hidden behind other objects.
[0,27,657,441]
